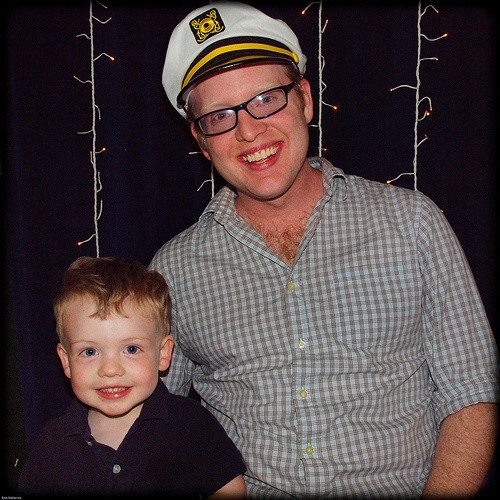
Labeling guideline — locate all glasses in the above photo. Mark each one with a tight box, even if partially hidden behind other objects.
[193,80,297,137]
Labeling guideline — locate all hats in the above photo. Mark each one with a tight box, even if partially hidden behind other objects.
[161,3,307,120]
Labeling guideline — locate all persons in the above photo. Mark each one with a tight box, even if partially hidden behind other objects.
[17,256,248,500]
[146,1,500,500]
